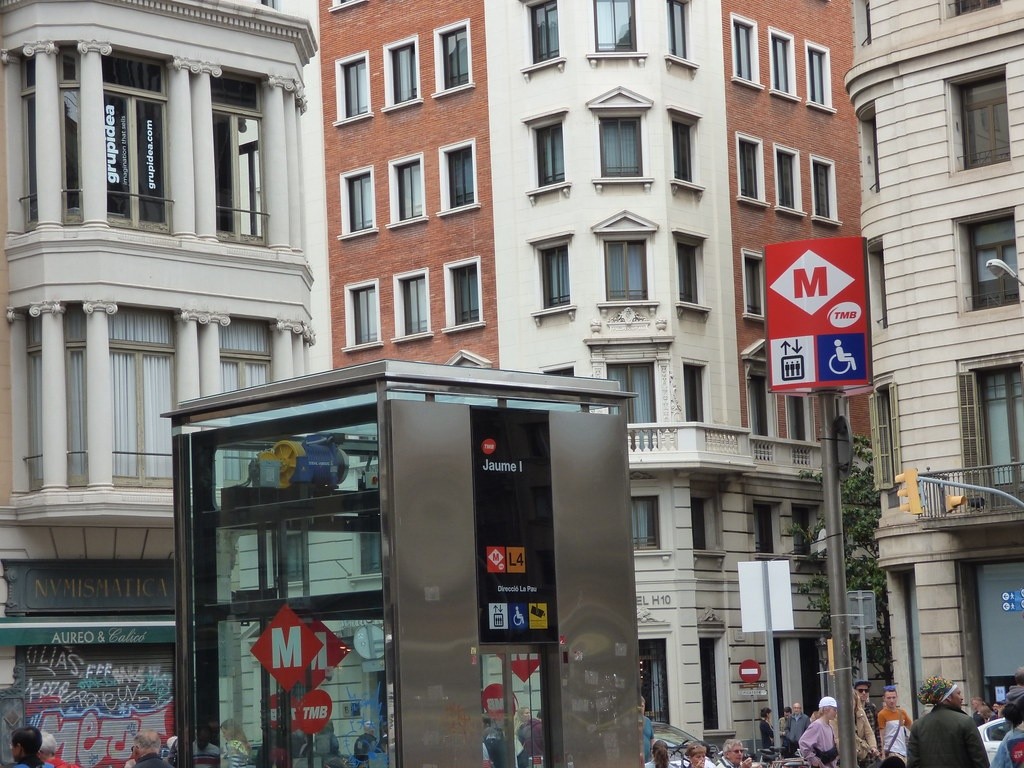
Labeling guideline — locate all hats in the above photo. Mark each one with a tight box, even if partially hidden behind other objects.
[917,675,957,705]
[819,696,837,708]
[854,680,872,689]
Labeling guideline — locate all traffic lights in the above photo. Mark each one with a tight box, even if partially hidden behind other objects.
[945,494,967,513]
[894,467,924,516]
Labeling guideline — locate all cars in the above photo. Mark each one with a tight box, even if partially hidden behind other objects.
[651,721,712,768]
[977,718,1007,766]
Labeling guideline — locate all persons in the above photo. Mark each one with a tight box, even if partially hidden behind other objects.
[904,676,991,768]
[39,731,81,768]
[9,727,56,768]
[969,666,1024,725]
[640,696,840,768]
[991,696,1024,768]
[853,680,913,768]
[125,706,543,768]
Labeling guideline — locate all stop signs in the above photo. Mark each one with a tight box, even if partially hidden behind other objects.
[739,659,761,684]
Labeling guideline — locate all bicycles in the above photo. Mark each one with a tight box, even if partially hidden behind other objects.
[670,739,811,768]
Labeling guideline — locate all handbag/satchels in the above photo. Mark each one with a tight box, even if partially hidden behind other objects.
[813,725,839,764]
[885,749,890,757]
[515,734,525,757]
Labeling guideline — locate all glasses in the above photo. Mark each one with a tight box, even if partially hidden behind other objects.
[855,689,870,693]
[729,750,743,753]
